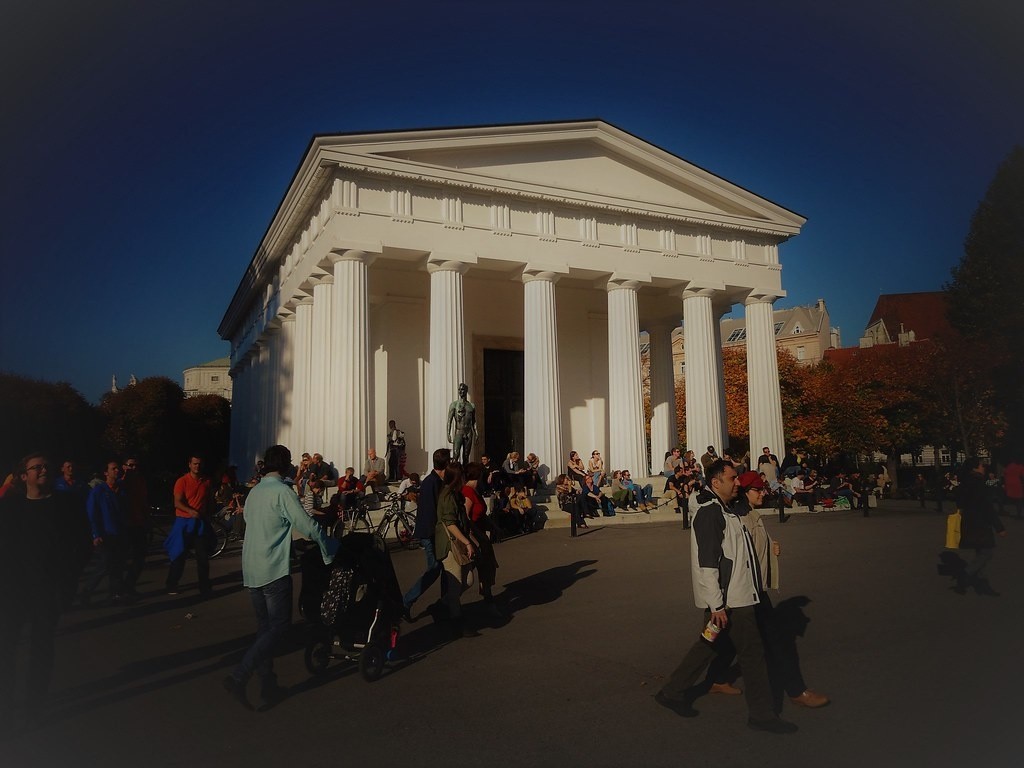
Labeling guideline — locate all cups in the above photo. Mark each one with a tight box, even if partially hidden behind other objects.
[529,489,535,496]
[699,605,731,645]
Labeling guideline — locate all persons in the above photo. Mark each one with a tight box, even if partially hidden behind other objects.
[442,465,505,621]
[446,382,478,468]
[0,451,80,731]
[87,461,129,606]
[212,416,424,540]
[164,454,222,598]
[955,471,1009,597]
[225,445,347,716]
[707,471,832,708]
[397,447,473,623]
[663,437,1023,572]
[655,461,798,734]
[472,447,658,538]
[0,456,154,596]
[428,462,482,639]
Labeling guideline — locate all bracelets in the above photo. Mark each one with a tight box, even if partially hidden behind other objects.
[465,542,470,546]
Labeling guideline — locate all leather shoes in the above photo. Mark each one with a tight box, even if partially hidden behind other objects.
[748,706,800,734]
[654,686,701,718]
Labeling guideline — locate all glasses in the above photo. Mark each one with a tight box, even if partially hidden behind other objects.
[124,463,137,468]
[25,464,50,471]
[745,487,765,494]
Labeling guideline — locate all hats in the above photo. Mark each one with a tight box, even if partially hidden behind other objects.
[738,470,766,489]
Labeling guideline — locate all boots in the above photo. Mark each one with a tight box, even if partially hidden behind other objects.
[953,570,970,595]
[980,577,1000,597]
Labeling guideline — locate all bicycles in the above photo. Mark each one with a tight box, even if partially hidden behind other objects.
[331,491,422,552]
[146,504,228,559]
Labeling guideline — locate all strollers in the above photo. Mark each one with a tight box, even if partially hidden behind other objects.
[301,532,409,685]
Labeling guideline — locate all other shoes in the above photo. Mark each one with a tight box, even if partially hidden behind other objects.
[397,601,414,624]
[221,675,252,708]
[790,689,830,707]
[701,678,742,695]
[447,622,480,638]
[260,672,278,698]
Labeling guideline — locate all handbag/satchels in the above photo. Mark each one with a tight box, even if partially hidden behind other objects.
[441,521,474,565]
[945,508,961,550]
